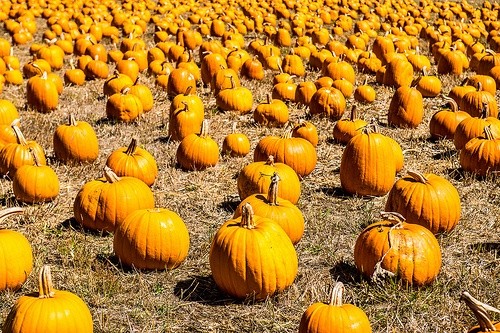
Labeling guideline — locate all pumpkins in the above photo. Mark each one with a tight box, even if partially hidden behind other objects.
[0,0,500,333]
